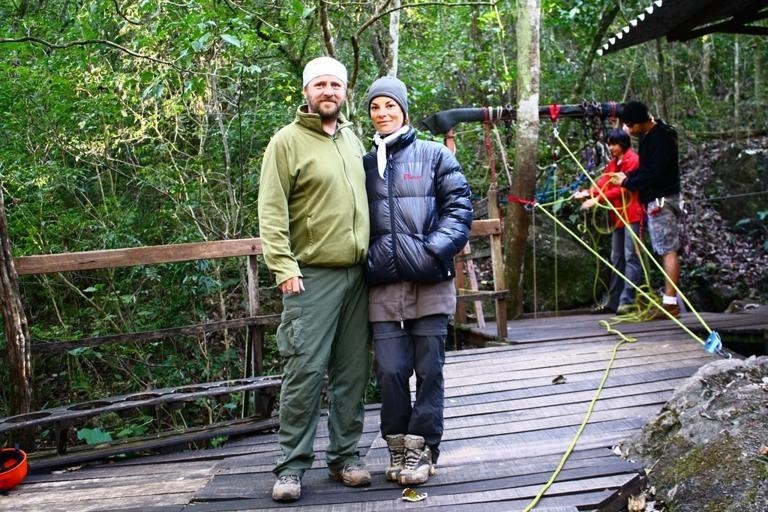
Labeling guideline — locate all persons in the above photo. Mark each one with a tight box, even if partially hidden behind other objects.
[258,56,371,502]
[574,128,648,315]
[609,101,681,320]
[362,75,473,486]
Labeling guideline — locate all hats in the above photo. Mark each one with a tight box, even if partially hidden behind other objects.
[368,76,408,117]
[302,57,347,87]
[621,102,648,121]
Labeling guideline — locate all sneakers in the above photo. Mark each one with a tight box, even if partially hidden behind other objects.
[650,305,680,320]
[272,475,302,501]
[591,305,628,315]
[386,434,435,484]
[328,463,371,487]
